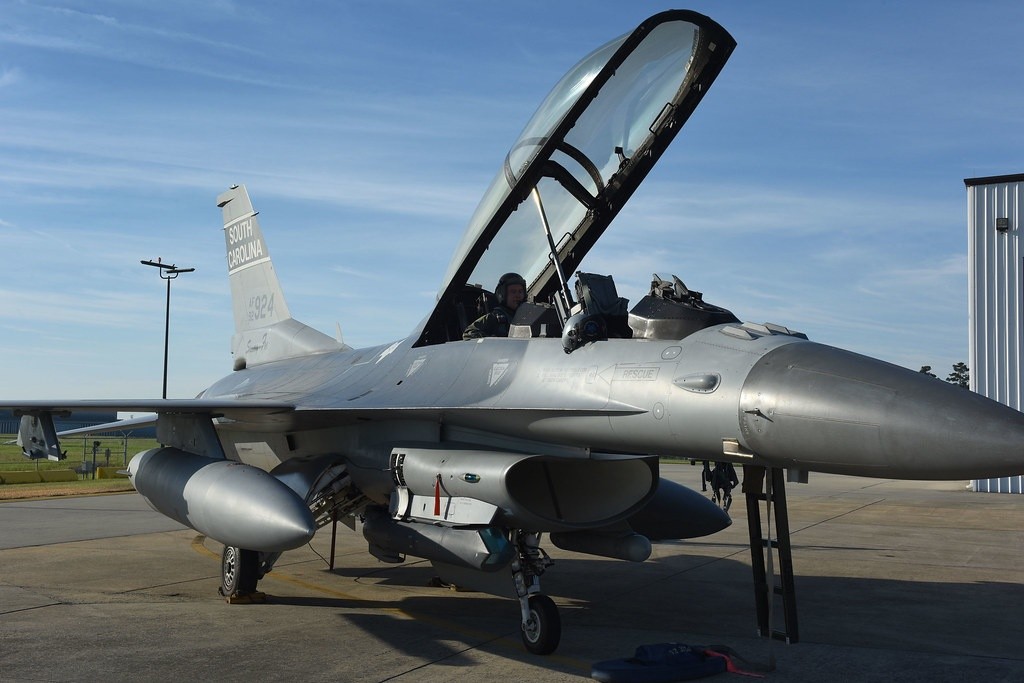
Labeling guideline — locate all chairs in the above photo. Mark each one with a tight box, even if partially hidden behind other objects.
[574,270,633,339]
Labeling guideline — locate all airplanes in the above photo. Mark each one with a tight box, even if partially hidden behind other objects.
[3,8,1024,658]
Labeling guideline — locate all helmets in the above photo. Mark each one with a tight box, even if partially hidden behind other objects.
[495,272,527,305]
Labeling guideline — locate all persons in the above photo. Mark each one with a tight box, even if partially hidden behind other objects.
[462,273,541,340]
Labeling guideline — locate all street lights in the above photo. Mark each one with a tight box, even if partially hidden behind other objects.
[141,257,196,400]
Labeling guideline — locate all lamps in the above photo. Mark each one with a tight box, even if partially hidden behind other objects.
[996,218,1009,233]
